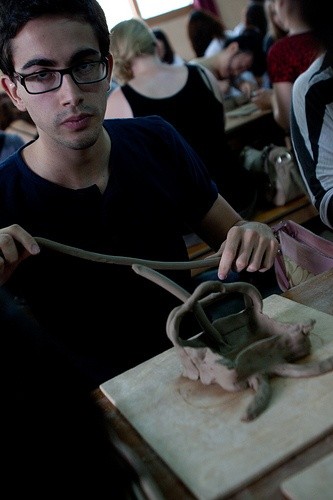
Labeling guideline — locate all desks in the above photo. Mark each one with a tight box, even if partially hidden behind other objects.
[226,101,271,142]
[186,194,316,277]
[91,269,333,500]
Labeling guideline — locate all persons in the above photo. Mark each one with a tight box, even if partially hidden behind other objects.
[151,0,333,134]
[104,19,226,173]
[290,47,333,229]
[0,0,279,500]
[186,31,257,100]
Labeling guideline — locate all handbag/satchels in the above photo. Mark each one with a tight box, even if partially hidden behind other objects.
[250,144,306,206]
[273,219,333,293]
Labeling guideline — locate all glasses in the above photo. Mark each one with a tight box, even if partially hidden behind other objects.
[13,55,109,95]
